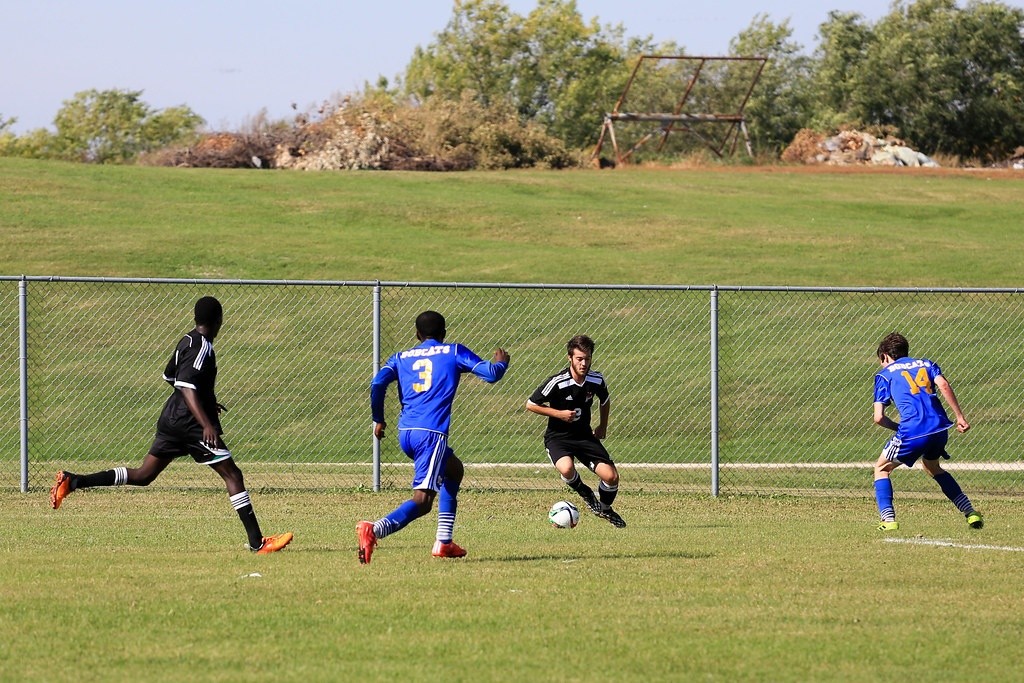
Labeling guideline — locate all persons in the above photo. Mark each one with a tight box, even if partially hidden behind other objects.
[51,297,293,553]
[356,311,511,564]
[873,333,983,532]
[526,335,628,528]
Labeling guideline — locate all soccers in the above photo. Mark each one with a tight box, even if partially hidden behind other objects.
[547,500,581,530]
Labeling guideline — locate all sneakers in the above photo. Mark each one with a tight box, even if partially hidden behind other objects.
[50,470,77,509]
[355,521,378,565]
[585,502,627,528]
[432,540,468,558]
[871,520,899,532]
[967,511,985,529]
[250,532,293,555]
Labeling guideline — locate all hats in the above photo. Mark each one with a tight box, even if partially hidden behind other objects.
[194,296,223,326]
